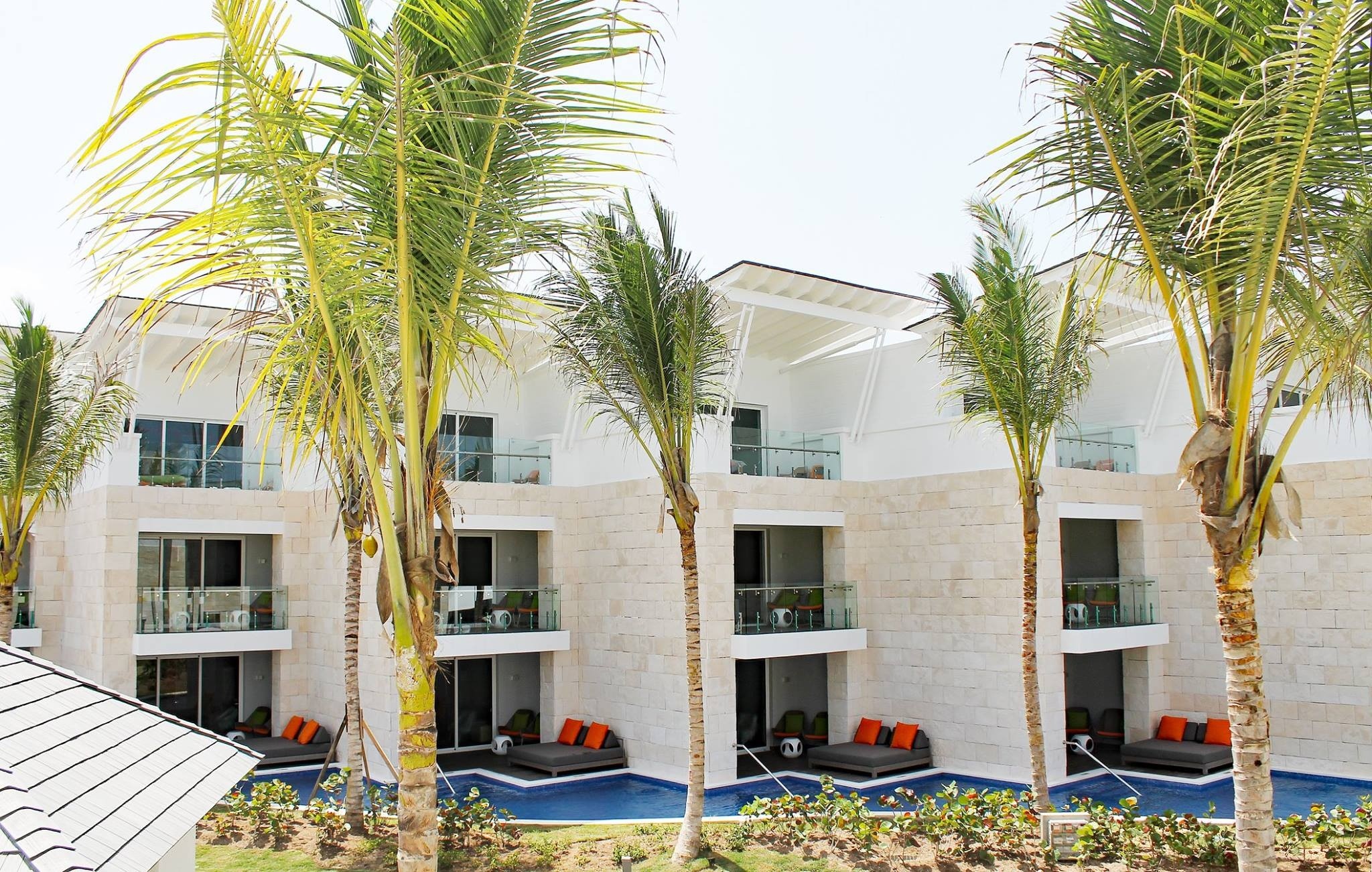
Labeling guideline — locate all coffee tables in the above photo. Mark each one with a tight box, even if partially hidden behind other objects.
[489,610,511,629]
[1070,734,1094,756]
[781,737,803,759]
[491,735,513,756]
[1065,604,1087,623]
[226,731,246,740]
[770,608,792,628]
[170,609,251,630]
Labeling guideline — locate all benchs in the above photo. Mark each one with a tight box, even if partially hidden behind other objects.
[807,727,932,778]
[231,723,334,770]
[507,727,627,778]
[1120,722,1232,775]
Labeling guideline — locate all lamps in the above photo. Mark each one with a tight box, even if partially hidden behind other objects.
[1041,812,1091,861]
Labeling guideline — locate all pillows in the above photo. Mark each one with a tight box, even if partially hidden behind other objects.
[246,712,319,744]
[505,591,523,607]
[809,588,822,607]
[512,712,609,749]
[1069,711,1231,745]
[777,592,798,606]
[529,594,539,608]
[785,713,920,752]
[1065,585,1117,602]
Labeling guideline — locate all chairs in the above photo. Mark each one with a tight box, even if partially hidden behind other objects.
[1090,584,1120,623]
[766,585,823,625]
[491,587,539,627]
[1062,581,1088,624]
[520,712,541,746]
[249,592,274,623]
[498,708,534,746]
[512,470,540,485]
[731,459,823,478]
[1071,459,1114,470]
[234,706,272,738]
[771,708,828,753]
[1065,707,1124,753]
